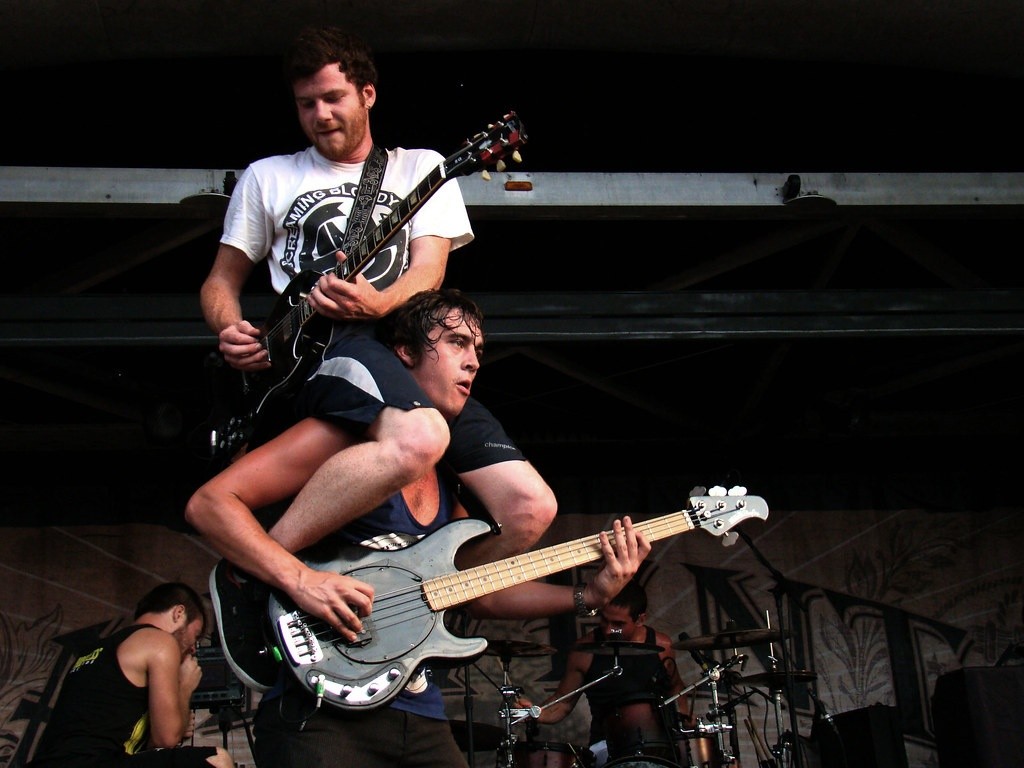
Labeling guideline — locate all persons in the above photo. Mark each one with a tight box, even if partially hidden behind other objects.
[31,580,235,768]
[508,575,701,768]
[184,290,653,766]
[177,26,565,693]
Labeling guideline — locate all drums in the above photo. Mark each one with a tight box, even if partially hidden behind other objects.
[510,740,597,768]
[671,728,722,768]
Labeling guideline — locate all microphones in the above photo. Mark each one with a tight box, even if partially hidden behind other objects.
[678,632,709,671]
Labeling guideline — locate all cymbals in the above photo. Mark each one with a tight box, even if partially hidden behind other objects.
[448,718,511,752]
[577,639,664,658]
[486,638,557,661]
[733,668,818,685]
[667,627,776,651]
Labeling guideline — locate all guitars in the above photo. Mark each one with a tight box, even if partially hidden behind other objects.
[190,484,773,722]
[207,111,531,470]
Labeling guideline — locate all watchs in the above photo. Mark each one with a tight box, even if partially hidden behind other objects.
[572,582,601,618]
